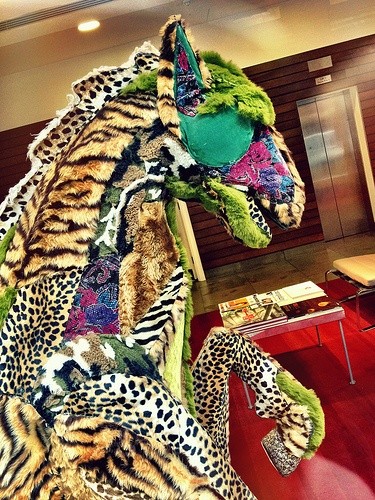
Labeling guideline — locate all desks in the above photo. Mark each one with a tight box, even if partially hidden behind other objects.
[218,279,356,410]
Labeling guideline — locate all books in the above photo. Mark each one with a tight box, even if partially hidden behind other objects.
[217,280,340,334]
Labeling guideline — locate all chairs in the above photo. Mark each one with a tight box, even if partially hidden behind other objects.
[325,253,375,333]
